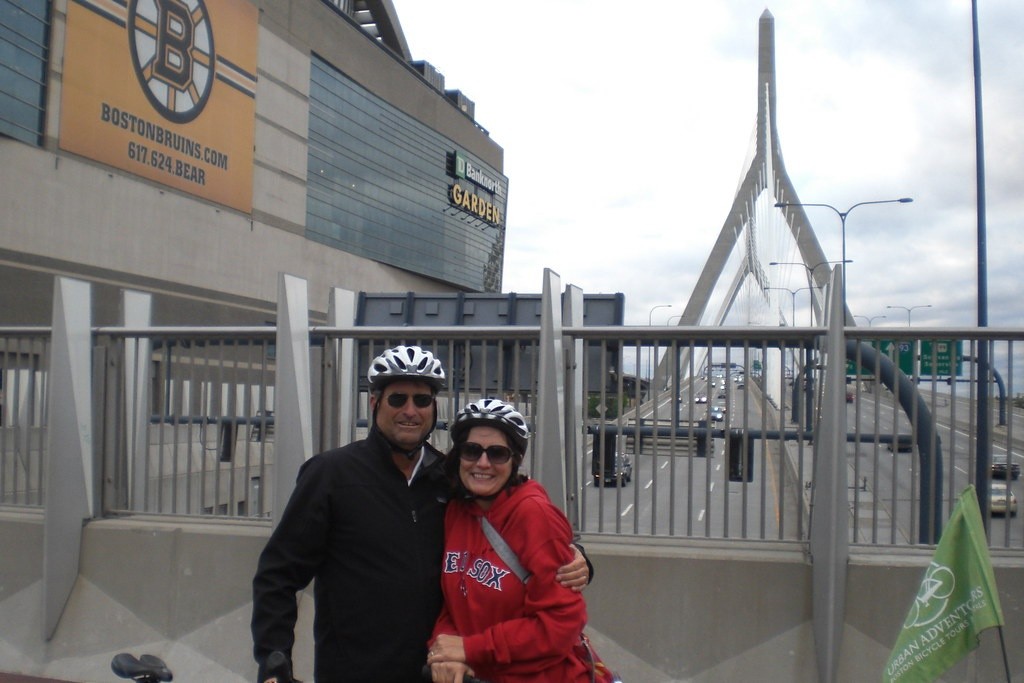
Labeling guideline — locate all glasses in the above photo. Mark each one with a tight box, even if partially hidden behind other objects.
[381,393,434,408]
[458,442,515,465]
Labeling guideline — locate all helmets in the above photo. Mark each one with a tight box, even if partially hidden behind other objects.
[451,399,528,457]
[367,345,446,394]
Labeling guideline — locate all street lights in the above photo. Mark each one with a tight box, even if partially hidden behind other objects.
[886,305,931,327]
[773,198,916,325]
[852,313,887,326]
[765,285,825,389]
[769,258,854,326]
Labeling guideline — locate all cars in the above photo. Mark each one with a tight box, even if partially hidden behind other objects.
[992,454,1020,479]
[670,372,745,421]
[594,450,633,489]
[886,432,914,454]
[991,482,1018,517]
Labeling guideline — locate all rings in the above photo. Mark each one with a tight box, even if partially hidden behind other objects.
[432,651,434,656]
[583,576,587,580]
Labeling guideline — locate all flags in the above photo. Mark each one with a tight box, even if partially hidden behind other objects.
[882,485,1004,683]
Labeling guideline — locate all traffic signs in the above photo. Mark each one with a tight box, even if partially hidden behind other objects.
[871,338,914,378]
[920,339,964,377]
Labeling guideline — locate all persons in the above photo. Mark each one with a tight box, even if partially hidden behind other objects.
[425,398,615,683]
[252,347,595,683]
